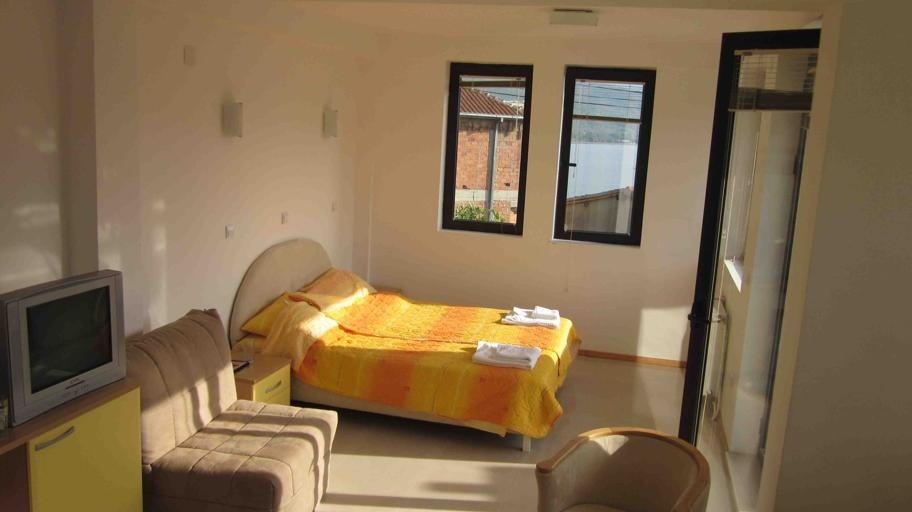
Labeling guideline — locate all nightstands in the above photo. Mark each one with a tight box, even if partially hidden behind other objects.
[228,351,292,406]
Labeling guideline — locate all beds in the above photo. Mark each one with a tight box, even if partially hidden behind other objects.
[231,238,583,453]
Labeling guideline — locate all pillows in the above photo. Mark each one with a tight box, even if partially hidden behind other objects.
[242,293,291,339]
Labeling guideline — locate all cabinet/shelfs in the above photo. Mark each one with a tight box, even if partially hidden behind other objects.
[1,383,145,512]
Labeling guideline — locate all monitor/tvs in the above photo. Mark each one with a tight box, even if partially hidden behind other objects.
[0,267,129,428]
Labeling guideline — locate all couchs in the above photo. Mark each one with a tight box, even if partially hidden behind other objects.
[123,310,340,512]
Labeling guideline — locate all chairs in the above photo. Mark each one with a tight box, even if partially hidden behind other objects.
[536,426,711,512]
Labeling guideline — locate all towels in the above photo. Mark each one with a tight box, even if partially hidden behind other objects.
[469,340,540,371]
[499,303,563,331]
